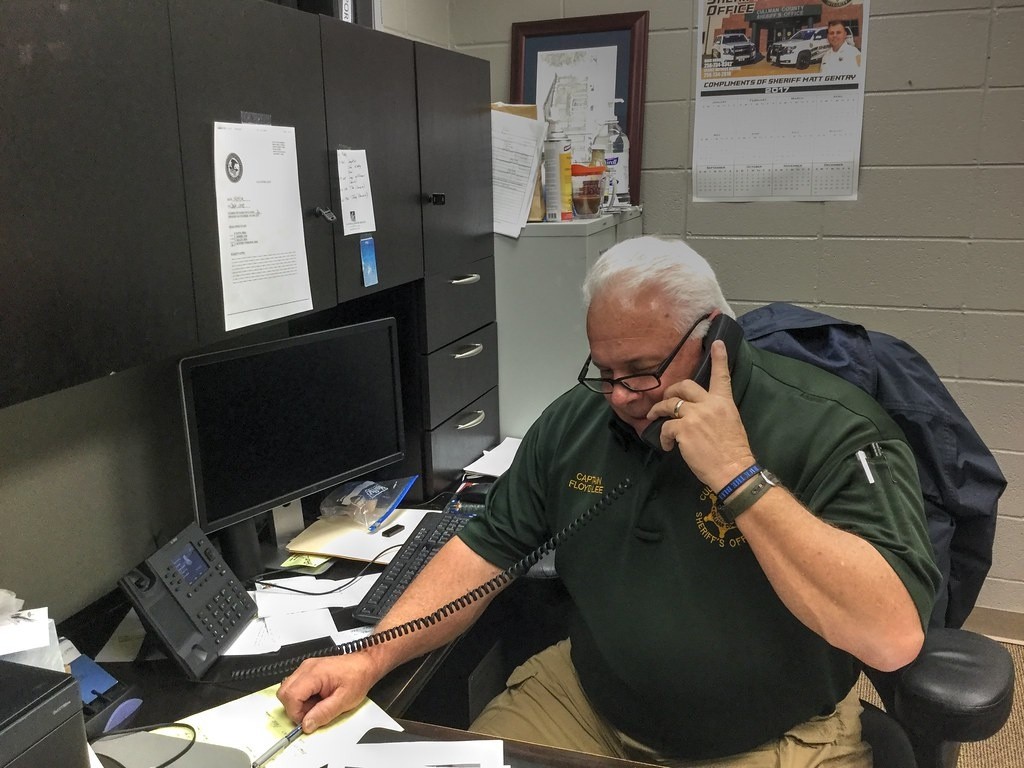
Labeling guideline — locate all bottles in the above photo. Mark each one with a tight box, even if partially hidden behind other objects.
[588,117,632,212]
[542,129,574,223]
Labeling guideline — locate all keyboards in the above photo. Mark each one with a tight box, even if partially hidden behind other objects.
[351,511,472,629]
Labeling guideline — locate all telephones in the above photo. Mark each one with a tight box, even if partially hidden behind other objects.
[642,314,745,456]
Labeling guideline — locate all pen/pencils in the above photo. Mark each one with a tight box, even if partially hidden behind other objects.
[253,723,303,768]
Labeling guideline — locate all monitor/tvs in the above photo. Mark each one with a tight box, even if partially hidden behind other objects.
[177,317,407,576]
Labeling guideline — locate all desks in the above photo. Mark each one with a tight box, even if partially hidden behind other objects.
[53,586,666,768]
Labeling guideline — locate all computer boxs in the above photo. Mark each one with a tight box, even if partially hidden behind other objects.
[440,635,507,729]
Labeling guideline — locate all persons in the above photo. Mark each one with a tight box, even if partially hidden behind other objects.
[276,237,942,766]
[820,20,861,72]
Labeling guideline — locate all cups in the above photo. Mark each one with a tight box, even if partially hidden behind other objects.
[571,161,616,218]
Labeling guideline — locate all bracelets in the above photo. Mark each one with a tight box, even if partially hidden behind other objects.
[719,470,781,525]
[714,463,762,505]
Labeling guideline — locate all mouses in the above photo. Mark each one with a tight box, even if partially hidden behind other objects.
[458,483,494,505]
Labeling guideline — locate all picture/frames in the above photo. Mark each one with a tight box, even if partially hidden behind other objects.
[510,10,649,206]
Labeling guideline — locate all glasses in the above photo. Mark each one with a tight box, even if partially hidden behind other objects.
[576,312,715,393]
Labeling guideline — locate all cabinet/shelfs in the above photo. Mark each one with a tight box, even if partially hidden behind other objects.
[0,1,501,501]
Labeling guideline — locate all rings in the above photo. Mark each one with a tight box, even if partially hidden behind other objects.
[673,399,687,420]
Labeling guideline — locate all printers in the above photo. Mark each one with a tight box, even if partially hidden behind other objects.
[0,659,91,768]
